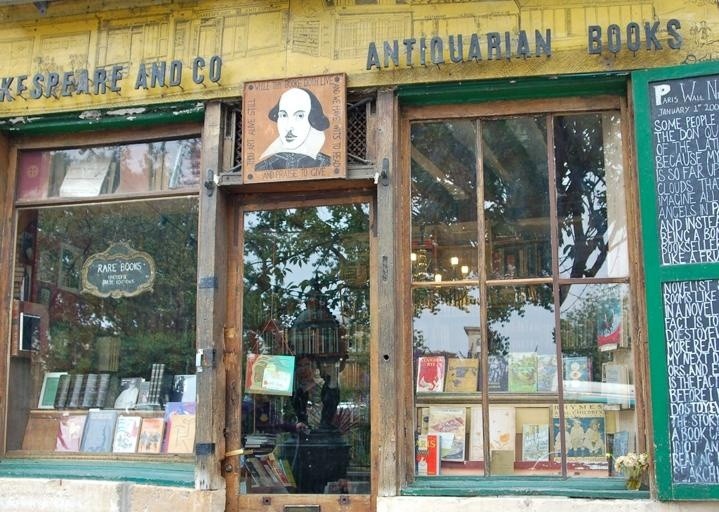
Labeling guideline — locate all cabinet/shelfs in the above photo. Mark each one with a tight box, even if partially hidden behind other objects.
[242,320,370,427]
[412,220,552,308]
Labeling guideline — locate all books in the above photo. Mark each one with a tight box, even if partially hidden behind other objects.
[15,150,55,198]
[12,243,87,360]
[57,137,201,197]
[38,364,196,456]
[245,293,370,493]
[94,335,121,373]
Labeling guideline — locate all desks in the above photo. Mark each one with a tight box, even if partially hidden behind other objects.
[22,410,165,451]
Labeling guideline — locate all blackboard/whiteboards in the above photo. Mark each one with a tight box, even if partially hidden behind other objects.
[80,238,158,300]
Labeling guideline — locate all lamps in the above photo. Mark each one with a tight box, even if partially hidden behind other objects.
[410,224,468,288]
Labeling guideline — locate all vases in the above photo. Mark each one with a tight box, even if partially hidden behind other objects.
[626,469,643,490]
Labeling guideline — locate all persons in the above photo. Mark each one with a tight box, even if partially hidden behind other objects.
[258,88,329,161]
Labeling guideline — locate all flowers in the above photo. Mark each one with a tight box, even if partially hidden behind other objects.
[605,453,650,473]
